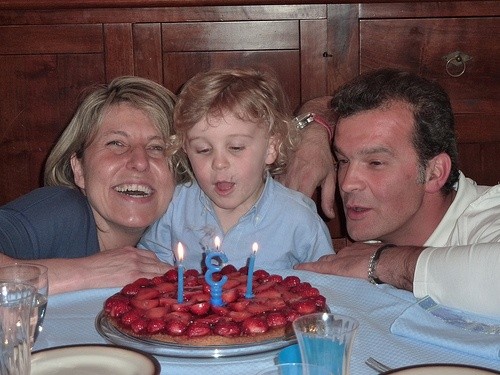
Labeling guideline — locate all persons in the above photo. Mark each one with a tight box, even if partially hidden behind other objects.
[272,68,500,318]
[135,66,337,270]
[0,76,179,297]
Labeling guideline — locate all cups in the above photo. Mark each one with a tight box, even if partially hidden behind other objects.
[292,311,358,375]
[0,262,48,348]
[0,283,32,375]
[256,362,333,375]
[279,338,347,375]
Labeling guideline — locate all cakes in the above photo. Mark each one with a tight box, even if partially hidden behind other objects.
[103,265,327,345]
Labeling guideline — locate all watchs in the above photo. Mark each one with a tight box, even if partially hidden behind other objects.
[294,112,334,145]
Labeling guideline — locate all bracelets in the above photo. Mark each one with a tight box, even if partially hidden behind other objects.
[368,243,396,284]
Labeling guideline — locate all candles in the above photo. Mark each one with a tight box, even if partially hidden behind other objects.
[242,242,258,298]
[204,235,228,306]
[177,241,184,303]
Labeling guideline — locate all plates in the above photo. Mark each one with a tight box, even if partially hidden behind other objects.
[377,363,500,375]
[28,343,162,375]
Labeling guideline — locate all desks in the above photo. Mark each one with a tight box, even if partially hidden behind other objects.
[0,269,500,375]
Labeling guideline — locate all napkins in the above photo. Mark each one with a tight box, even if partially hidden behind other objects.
[391,295,500,360]
